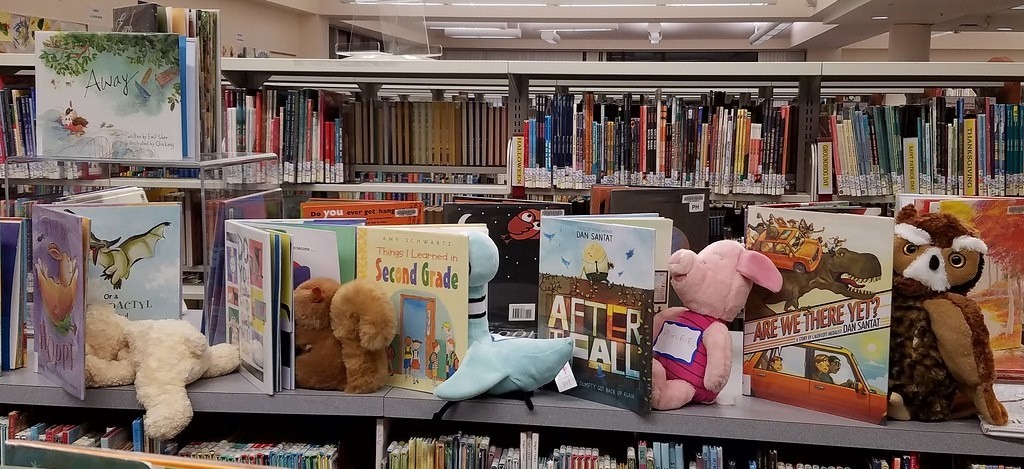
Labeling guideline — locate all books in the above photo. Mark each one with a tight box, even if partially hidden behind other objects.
[0,4,1024,469]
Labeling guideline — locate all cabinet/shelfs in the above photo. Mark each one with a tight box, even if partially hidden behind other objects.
[0,53,1024,469]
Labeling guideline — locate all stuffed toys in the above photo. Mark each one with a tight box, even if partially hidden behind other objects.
[83,305,241,440]
[886,204,1008,424]
[292,278,398,395]
[433,229,574,412]
[650,239,783,410]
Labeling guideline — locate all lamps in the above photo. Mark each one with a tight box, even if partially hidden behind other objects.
[747,22,795,46]
[647,22,663,44]
[422,21,618,45]
